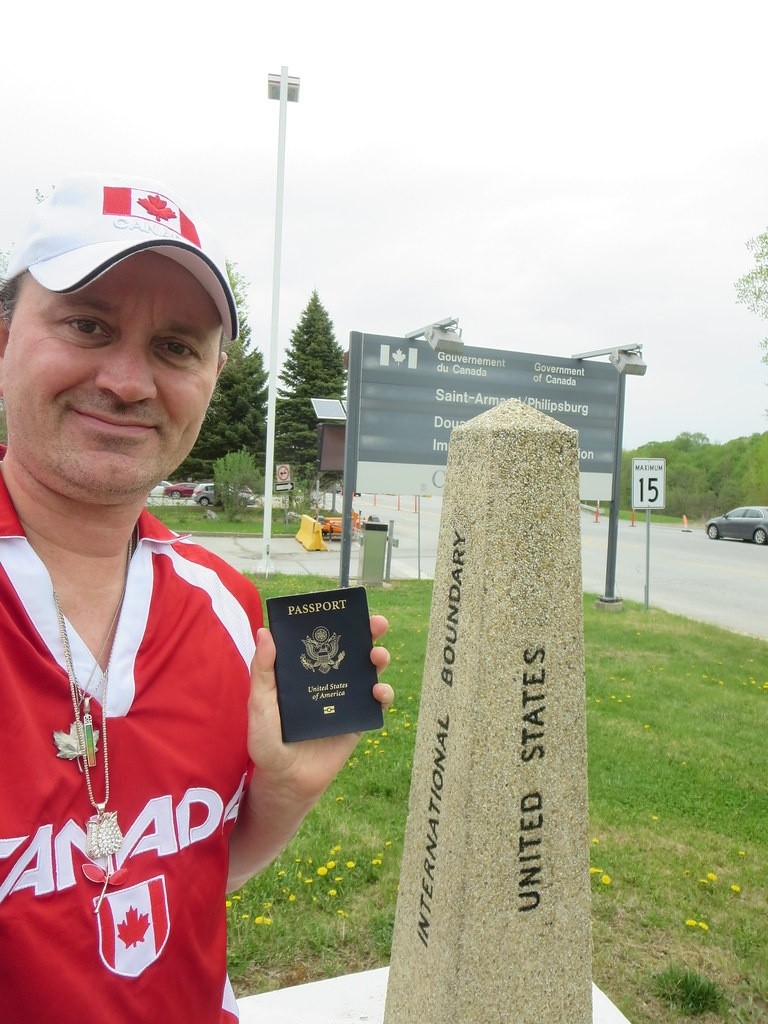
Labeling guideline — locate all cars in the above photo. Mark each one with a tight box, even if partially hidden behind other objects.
[192,483,255,508]
[148,481,173,497]
[705,506,768,545]
[163,482,198,499]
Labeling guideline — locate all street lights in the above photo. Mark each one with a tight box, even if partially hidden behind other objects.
[255,66,299,577]
[372,495,376,506]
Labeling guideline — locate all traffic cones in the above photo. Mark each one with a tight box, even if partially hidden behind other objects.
[593,504,600,523]
[414,496,418,513]
[681,515,692,532]
[397,496,402,511]
[629,510,636,527]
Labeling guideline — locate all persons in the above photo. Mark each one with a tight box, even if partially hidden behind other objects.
[0,185,395,1024]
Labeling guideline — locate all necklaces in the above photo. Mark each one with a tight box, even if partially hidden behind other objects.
[53,537,132,914]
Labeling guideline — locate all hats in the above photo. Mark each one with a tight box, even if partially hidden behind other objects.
[0,176,239,340]
